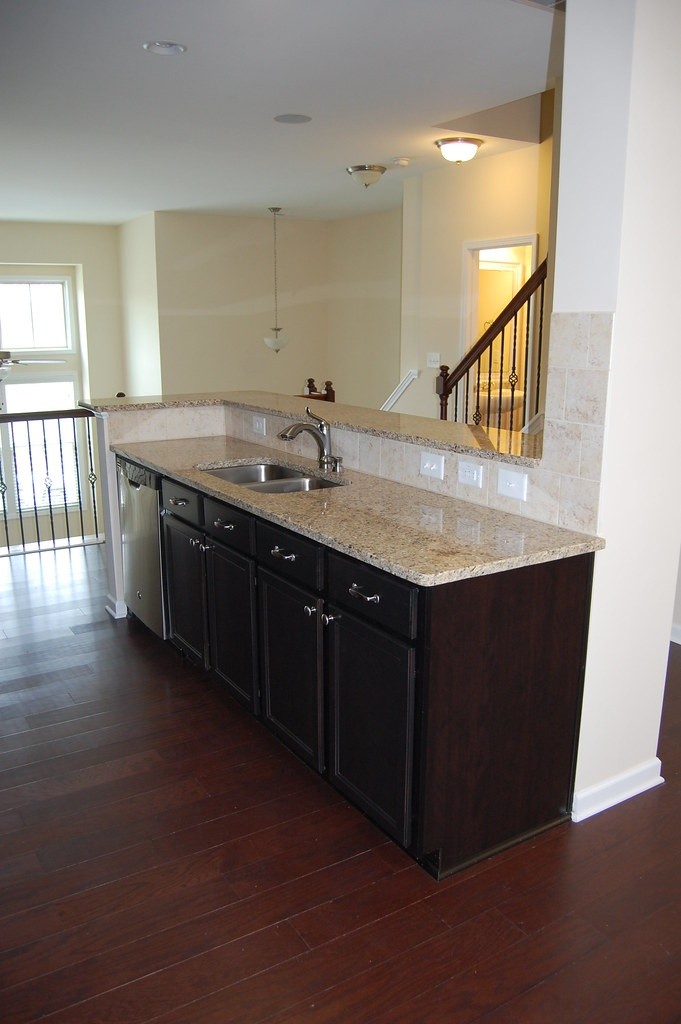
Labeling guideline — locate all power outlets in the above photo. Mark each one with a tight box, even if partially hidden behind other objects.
[253,417,266,435]
[458,462,482,489]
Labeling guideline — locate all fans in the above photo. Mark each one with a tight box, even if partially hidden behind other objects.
[0,348,66,369]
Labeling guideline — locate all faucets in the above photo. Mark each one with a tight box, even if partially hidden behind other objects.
[277,406,344,472]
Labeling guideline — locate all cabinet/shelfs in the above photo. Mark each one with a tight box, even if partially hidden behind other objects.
[252,512,598,876]
[161,479,259,706]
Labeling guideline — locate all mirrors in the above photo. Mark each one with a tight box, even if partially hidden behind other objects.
[478,260,524,390]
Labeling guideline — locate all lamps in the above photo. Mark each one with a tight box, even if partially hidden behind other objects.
[346,164,387,190]
[264,206,290,354]
[434,137,484,167]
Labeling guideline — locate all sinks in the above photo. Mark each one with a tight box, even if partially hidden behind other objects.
[248,477,342,492]
[200,465,303,485]
